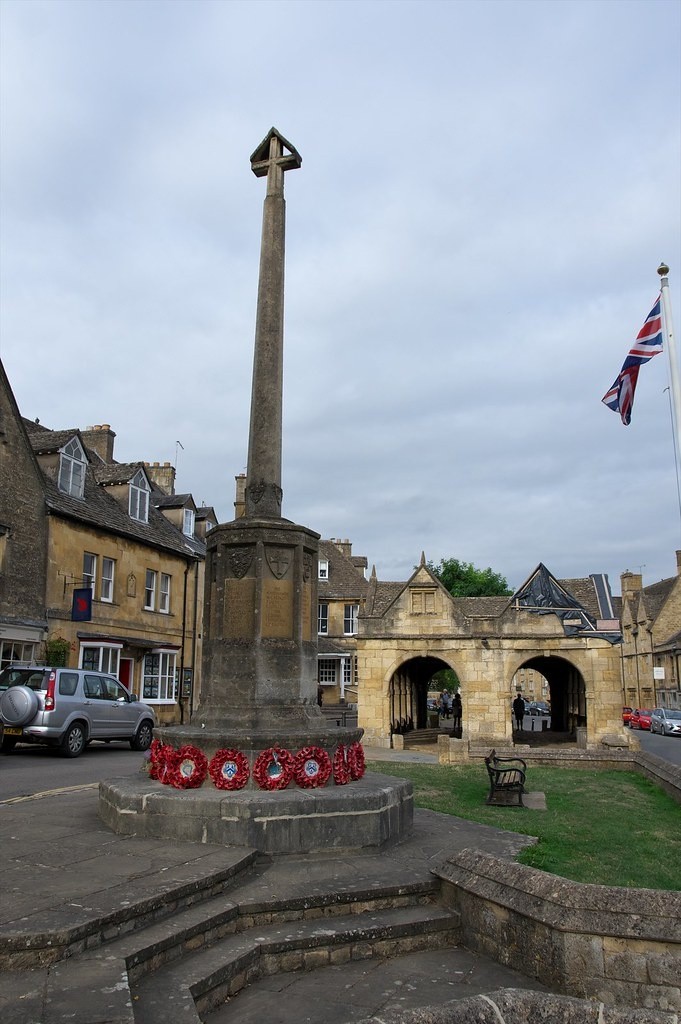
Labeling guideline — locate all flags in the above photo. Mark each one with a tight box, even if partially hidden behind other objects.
[601,293,666,426]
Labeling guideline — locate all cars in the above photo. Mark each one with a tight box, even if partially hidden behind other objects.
[447,699,453,714]
[427,697,437,711]
[622,705,633,722]
[510,700,530,716]
[650,708,681,736]
[628,707,654,731]
[529,701,551,716]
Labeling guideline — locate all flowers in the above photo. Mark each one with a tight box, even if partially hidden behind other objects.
[139,740,365,791]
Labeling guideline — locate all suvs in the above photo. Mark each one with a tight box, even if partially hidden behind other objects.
[0,664,158,758]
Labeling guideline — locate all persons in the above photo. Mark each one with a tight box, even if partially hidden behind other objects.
[513,693,525,731]
[437,688,462,734]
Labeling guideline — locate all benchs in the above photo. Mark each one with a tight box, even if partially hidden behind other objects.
[484,748,529,808]
